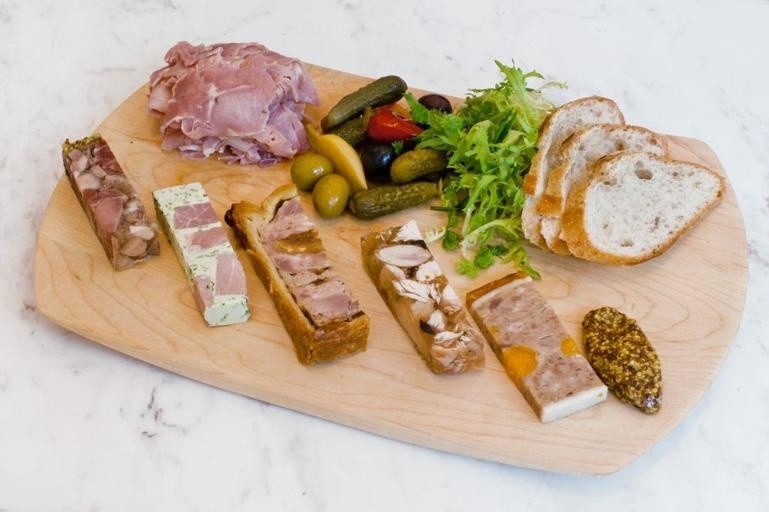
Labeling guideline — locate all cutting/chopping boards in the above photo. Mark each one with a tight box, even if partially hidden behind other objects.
[30,44,754,482]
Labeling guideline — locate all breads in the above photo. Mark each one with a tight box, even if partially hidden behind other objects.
[520,95,725,268]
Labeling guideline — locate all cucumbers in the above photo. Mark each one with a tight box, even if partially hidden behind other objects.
[321,75,451,221]
